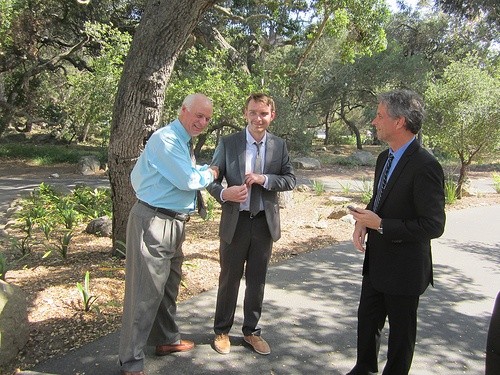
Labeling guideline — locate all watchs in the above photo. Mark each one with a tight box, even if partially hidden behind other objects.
[377,219,384,235]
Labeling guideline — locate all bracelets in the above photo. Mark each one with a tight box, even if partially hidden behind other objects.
[262,174,266,185]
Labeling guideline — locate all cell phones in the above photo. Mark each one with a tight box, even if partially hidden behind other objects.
[347,205,358,213]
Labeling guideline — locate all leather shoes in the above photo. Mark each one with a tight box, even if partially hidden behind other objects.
[243,335,271,354]
[156,339,195,356]
[119,369,146,375]
[214,333,230,354]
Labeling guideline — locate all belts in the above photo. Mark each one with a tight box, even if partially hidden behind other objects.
[138,199,190,222]
[239,210,266,219]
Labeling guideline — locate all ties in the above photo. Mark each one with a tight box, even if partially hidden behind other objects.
[372,153,395,213]
[189,139,207,220]
[250,141,263,216]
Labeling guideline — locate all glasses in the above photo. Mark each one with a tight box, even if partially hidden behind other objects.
[187,105,212,123]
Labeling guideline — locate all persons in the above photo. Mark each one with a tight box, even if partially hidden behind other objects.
[346,88,446,375]
[206,92,297,355]
[119,93,220,375]
